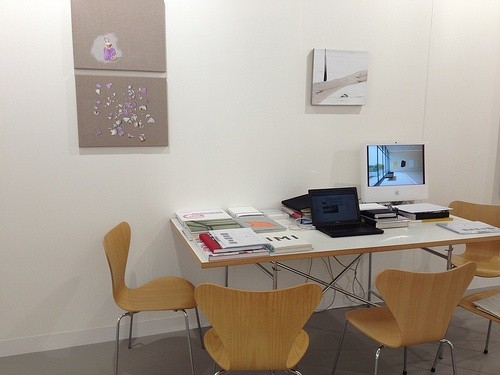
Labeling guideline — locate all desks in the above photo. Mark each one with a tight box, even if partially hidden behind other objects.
[170,215,500,361]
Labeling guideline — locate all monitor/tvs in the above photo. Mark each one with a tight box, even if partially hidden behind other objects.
[361,144,429,205]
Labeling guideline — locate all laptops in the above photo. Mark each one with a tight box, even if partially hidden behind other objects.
[308,187,384,238]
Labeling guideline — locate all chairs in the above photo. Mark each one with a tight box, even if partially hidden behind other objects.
[102,221,204,375]
[195,283,324,375]
[448,200,500,278]
[330,261,478,375]
[439,289,500,360]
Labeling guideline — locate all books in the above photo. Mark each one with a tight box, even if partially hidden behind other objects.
[174,201,500,261]
